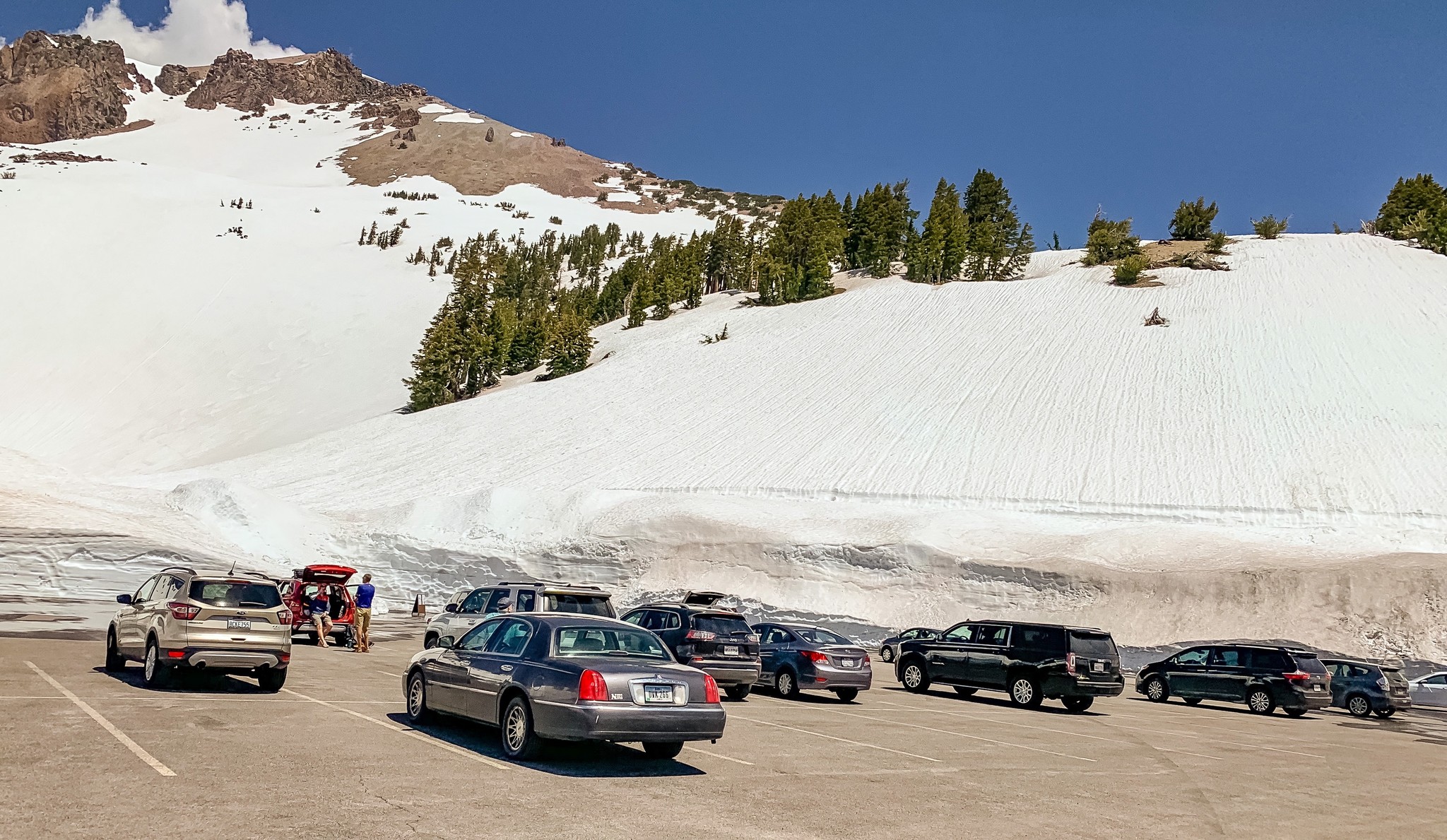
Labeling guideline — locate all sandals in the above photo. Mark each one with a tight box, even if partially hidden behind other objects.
[352,648,362,653]
[362,648,370,653]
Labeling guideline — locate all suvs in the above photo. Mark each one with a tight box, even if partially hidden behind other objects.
[104,566,294,694]
[1318,658,1412,718]
[275,563,357,647]
[895,618,1126,713]
[618,589,763,700]
[422,581,626,654]
[1134,641,1334,718]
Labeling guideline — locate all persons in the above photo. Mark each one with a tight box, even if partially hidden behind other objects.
[308,583,334,647]
[352,574,376,653]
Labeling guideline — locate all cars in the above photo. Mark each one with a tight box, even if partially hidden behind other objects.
[1408,671,1447,709]
[400,611,727,761]
[878,626,967,664]
[748,621,873,702]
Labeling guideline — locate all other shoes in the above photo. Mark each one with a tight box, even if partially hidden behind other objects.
[317,645,323,646]
[324,645,329,648]
